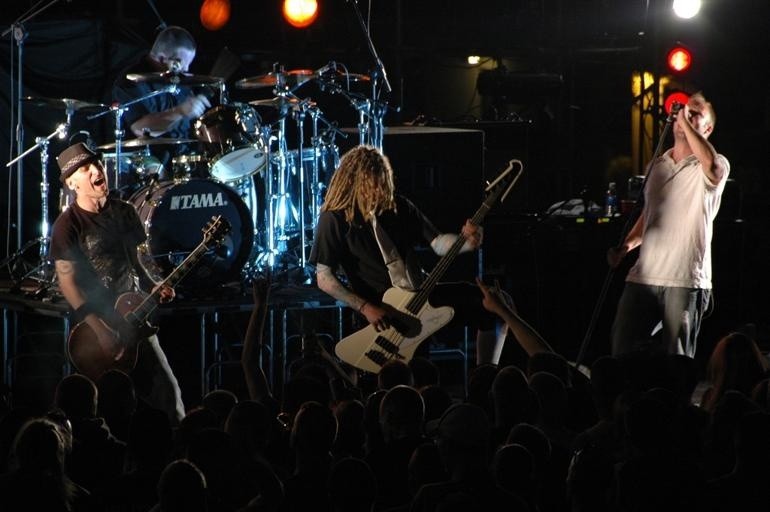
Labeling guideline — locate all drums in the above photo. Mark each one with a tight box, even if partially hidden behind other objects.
[125,177,253,302]
[193,104,267,184]
[269,144,339,232]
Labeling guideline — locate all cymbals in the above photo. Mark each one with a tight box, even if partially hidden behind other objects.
[249,96,317,108]
[96,136,195,148]
[20,96,104,110]
[127,71,222,85]
[234,70,316,86]
[308,72,369,81]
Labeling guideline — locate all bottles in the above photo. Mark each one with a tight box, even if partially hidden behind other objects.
[607,181,619,218]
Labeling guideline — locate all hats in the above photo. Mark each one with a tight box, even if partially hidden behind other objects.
[57,141,100,183]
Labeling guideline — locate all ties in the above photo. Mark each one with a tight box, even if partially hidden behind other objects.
[369,208,416,292]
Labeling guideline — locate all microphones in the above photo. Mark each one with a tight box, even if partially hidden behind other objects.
[169,73,182,94]
[670,101,685,112]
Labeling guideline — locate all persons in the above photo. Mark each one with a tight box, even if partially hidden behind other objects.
[308,142,516,364]
[50,142,186,424]
[608,93,731,358]
[105,26,212,168]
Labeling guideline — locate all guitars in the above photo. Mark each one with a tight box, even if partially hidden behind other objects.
[333,158,524,377]
[67,213,233,380]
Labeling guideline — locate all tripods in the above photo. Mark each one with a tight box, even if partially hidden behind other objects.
[9,140,62,294]
[244,100,387,279]
